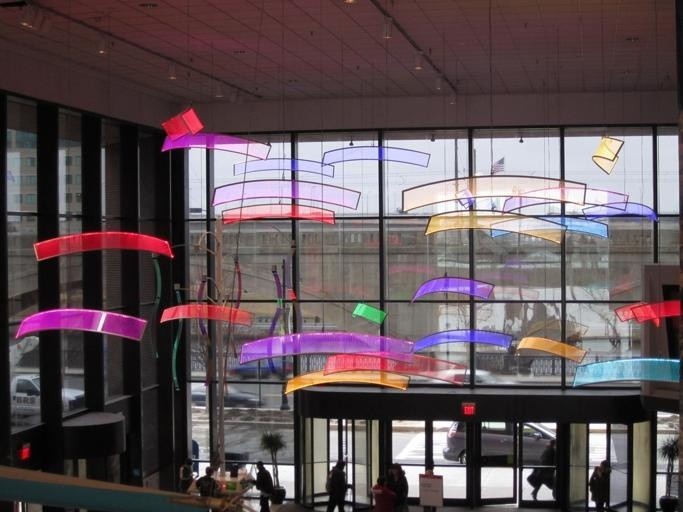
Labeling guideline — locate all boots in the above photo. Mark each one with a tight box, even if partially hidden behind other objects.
[11,372,86,415]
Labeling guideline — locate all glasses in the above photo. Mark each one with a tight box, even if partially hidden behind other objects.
[15,4,226,100]
[382,14,459,108]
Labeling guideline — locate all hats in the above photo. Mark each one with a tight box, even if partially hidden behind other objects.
[441,415,561,467]
[429,369,517,386]
[227,360,294,380]
[190,377,264,409]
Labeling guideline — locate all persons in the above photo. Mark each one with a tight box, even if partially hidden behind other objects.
[594,459,611,512]
[384,463,409,512]
[526,438,556,500]
[371,475,397,512]
[249,459,274,511]
[323,459,349,511]
[178,458,194,494]
[195,466,221,497]
[589,465,604,512]
